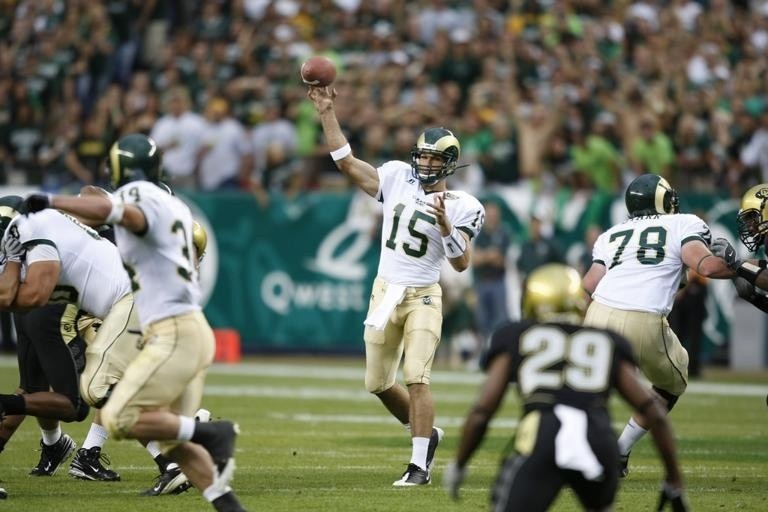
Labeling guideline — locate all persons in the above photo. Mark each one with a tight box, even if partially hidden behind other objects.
[516,210,557,269]
[580,173,768,479]
[709,184,768,311]
[473,204,509,338]
[672,271,707,381]
[14,134,249,512]
[443,264,691,512]
[0,1,768,205]
[308,84,485,489]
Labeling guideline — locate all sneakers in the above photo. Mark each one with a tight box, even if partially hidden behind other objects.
[425,424,445,470]
[618,448,632,479]
[27,432,77,477]
[67,446,121,482]
[202,418,241,491]
[392,462,432,487]
[140,461,195,497]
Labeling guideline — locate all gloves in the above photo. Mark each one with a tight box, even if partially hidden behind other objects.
[441,463,468,502]
[709,237,736,266]
[19,191,54,218]
[656,473,693,512]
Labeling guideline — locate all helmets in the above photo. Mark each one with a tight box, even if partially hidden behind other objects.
[192,218,208,262]
[624,172,681,216]
[410,127,471,184]
[104,132,163,192]
[736,182,768,252]
[520,262,588,321]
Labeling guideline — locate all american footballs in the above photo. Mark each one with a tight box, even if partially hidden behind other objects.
[299,55,337,88]
